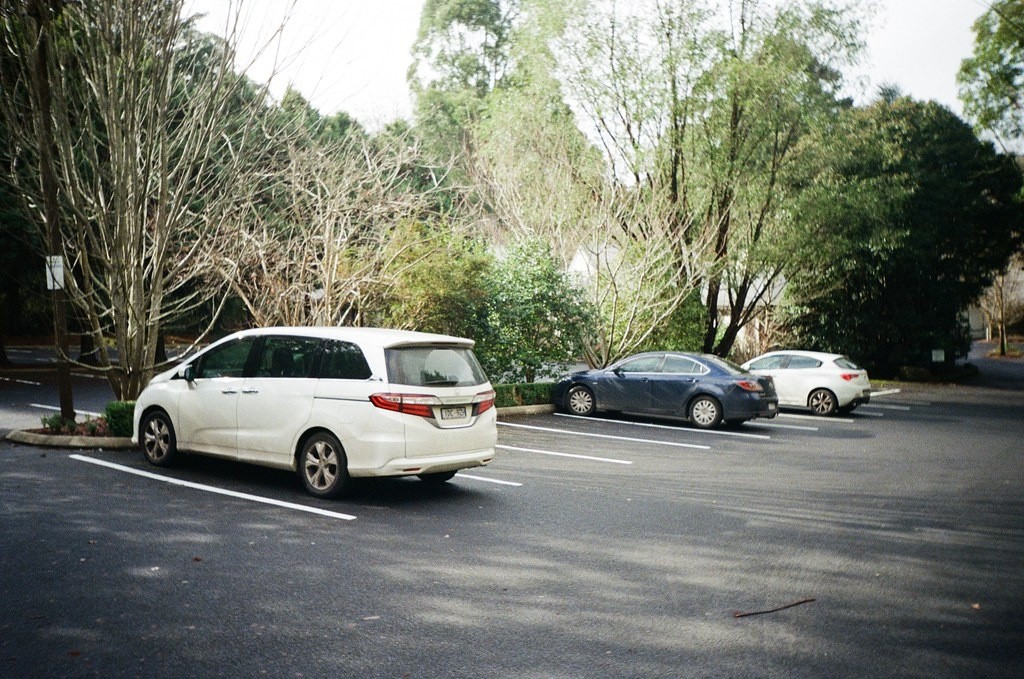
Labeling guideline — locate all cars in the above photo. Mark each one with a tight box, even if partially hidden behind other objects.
[555,352,779,428]
[739,351,873,415]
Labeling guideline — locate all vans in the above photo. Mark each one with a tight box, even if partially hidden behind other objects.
[131,325,498,497]
